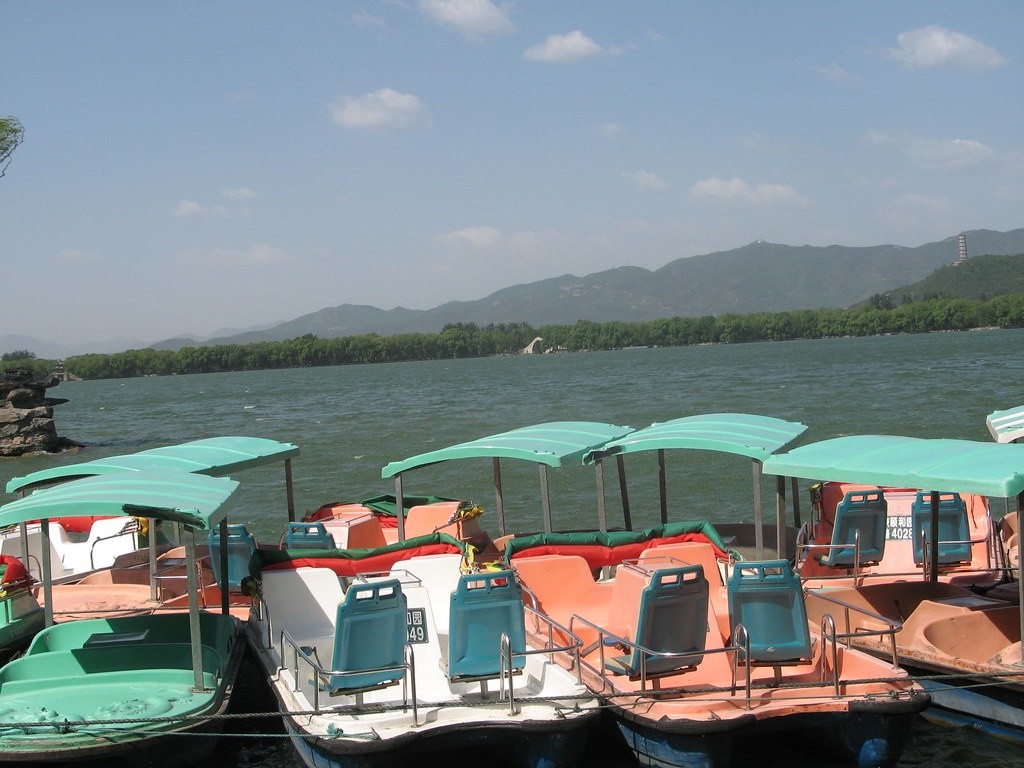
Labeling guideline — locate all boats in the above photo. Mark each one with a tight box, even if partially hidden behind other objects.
[0,403,1024,768]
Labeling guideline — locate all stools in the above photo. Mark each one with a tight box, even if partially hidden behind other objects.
[83,629,151,648]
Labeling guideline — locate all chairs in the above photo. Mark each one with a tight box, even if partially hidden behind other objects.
[815,490,887,569]
[438,570,527,683]
[308,578,407,697]
[601,564,710,681]
[911,492,972,567]
[727,560,813,667]
[208,524,258,595]
[287,522,352,594]
